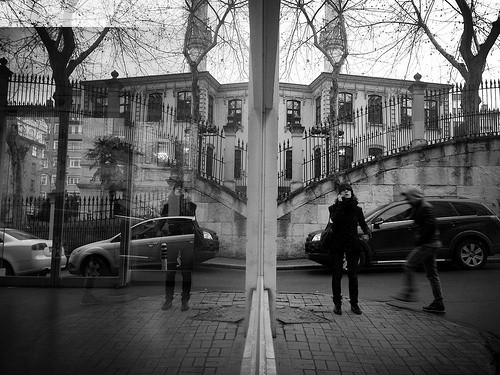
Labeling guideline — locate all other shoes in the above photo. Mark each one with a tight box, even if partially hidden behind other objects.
[160,299,173,310]
[334,303,342,315]
[351,304,362,314]
[180,297,190,311]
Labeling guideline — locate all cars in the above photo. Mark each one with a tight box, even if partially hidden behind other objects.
[0,228,67,276]
[69,216,220,275]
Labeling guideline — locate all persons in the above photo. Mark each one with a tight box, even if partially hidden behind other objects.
[154,175,204,313]
[328,183,374,315]
[387,186,447,314]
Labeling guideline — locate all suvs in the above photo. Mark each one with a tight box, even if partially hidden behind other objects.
[305,195,500,267]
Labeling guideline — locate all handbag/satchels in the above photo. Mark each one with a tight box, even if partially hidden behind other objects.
[320,213,337,249]
[190,213,205,254]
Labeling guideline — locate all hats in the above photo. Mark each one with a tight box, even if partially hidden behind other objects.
[401,188,424,200]
[174,179,188,193]
[338,183,352,192]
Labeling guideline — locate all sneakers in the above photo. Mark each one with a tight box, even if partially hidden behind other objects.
[423,300,445,313]
